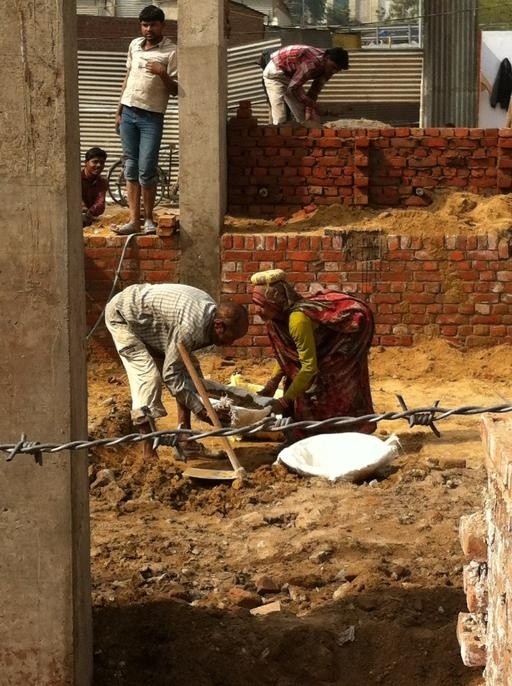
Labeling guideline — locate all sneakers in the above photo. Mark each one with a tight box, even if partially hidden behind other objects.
[173,448,226,462]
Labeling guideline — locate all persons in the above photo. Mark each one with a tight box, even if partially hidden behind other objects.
[81,148,107,227]
[110,5,178,235]
[105,282,249,461]
[263,44,348,126]
[250,268,375,442]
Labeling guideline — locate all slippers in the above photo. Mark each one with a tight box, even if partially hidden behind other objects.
[111,222,141,234]
[144,224,156,235]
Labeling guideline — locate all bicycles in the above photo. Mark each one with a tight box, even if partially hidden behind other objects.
[108,143,179,212]
